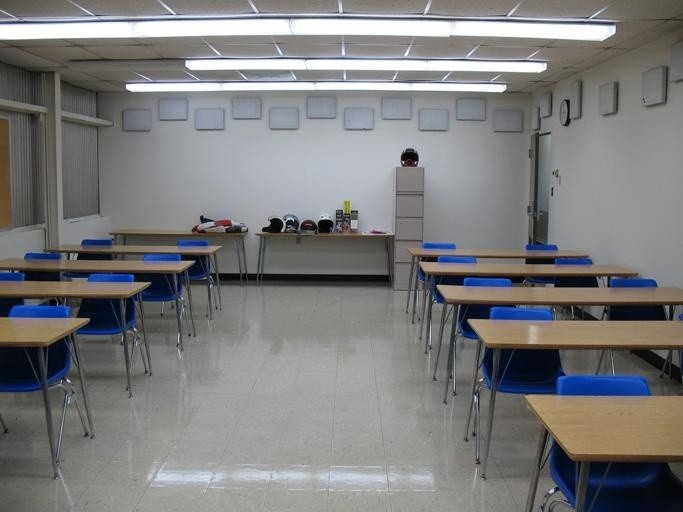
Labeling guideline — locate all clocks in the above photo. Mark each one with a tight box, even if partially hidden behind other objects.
[557,98,572,128]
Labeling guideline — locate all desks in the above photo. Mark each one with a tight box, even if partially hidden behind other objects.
[254,229,396,285]
[108,228,249,283]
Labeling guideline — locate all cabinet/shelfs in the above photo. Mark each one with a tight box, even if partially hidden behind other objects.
[393,166,425,291]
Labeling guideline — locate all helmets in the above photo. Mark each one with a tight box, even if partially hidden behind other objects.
[261,216,284,233]
[301,219,317,230]
[283,214,299,233]
[400,148,419,167]
[318,214,333,233]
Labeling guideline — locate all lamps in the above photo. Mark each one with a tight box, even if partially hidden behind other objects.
[0,11,622,50]
[68,54,554,76]
[120,80,512,98]
